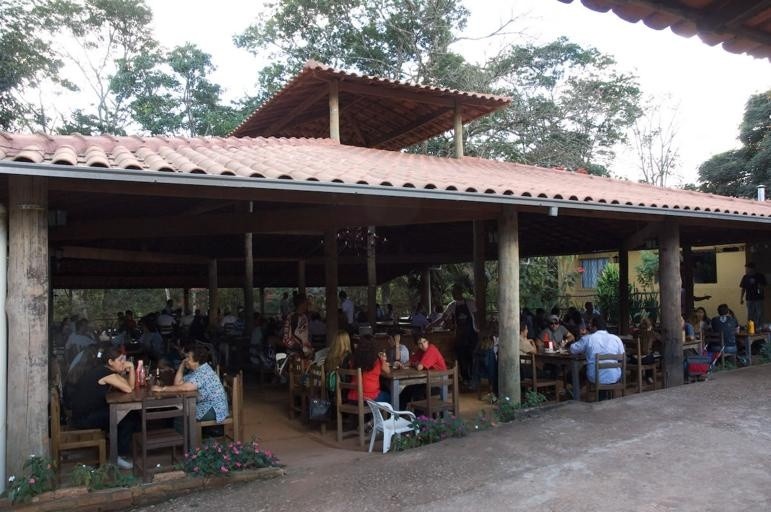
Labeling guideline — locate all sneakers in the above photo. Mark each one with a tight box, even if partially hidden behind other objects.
[116,456,132,471]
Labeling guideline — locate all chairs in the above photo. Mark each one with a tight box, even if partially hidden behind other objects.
[51,308,476,489]
[478,309,757,409]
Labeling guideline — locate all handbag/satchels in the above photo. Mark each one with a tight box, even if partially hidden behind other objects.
[308,398,331,422]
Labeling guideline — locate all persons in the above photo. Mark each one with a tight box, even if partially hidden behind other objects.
[56,286,738,471]
[740,261,770,331]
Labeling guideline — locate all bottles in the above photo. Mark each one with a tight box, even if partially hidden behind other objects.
[543,339,571,355]
[378,347,392,367]
[135,359,162,387]
